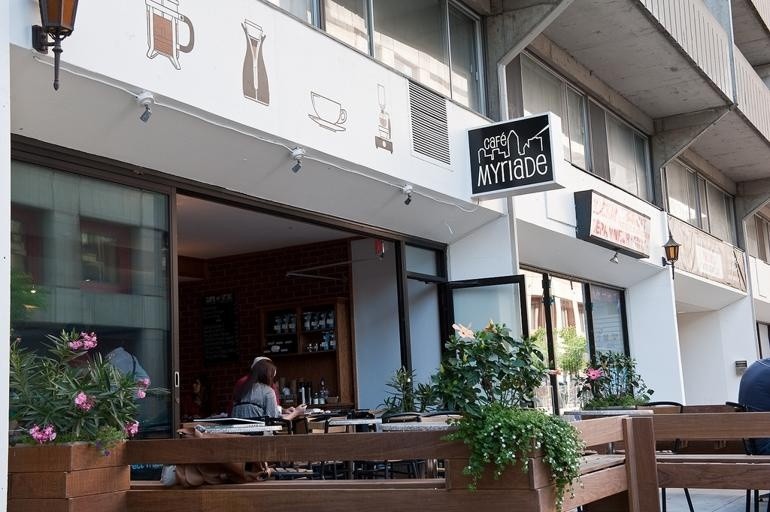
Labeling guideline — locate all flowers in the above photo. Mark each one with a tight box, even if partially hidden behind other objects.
[576,351,655,408]
[10,329,150,443]
[434,324,548,410]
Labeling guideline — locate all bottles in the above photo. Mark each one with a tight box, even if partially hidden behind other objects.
[298,376,328,406]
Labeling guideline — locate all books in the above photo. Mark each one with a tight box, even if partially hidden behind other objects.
[191,415,284,434]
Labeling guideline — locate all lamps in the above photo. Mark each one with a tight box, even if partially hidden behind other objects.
[289,147,307,173]
[136,91,155,123]
[32,1,79,89]
[403,185,413,205]
[662,231,682,279]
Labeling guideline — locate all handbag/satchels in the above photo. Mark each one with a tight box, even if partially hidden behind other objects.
[160,424,271,487]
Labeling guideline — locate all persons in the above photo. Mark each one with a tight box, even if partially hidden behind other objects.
[180,375,211,421]
[738,356,769,455]
[97,342,153,400]
[226,355,295,421]
[231,358,308,423]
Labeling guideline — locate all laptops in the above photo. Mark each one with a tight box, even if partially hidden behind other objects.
[193,416,265,428]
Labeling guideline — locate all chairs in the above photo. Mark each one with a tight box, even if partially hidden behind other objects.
[726,401,770,512]
[165,405,463,481]
[574,450,597,512]
[616,401,694,510]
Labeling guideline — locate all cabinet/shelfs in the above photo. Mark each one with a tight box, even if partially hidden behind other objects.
[256,295,353,410]
